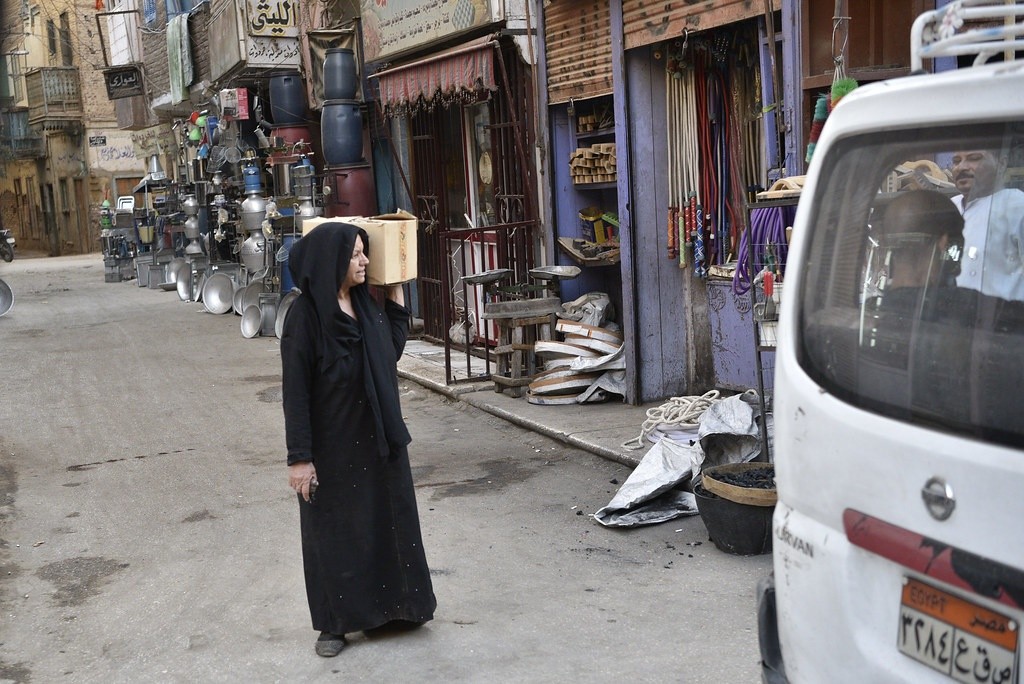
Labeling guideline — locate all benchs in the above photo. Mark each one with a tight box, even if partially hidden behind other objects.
[804,305,1024,432]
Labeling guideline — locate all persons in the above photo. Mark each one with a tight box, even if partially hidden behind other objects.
[950,149,1024,301]
[280,221,438,657]
[865,190,1024,425]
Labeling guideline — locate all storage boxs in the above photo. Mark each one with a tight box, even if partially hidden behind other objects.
[303,210,418,284]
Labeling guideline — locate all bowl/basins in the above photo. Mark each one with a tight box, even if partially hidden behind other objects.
[691,480,775,557]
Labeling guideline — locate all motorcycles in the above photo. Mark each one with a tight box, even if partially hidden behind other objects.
[0,227,17,263]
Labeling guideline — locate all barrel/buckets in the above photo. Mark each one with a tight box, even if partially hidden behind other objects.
[268,70,310,125]
[139,226,154,244]
[323,47,359,98]
[321,99,364,163]
[320,164,376,219]
[243,163,261,189]
[269,127,312,165]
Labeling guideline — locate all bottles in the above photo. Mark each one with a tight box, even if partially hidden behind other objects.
[100,208,111,228]
[181,193,200,215]
[184,214,200,239]
[102,200,111,209]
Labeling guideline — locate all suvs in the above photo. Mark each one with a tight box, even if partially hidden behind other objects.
[754,0,1023,684]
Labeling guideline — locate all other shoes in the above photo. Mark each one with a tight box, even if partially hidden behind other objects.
[316,632,344,657]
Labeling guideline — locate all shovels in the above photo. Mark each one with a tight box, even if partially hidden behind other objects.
[763,271,777,319]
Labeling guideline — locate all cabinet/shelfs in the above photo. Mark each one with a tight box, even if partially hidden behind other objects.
[567,94,617,190]
[745,188,963,463]
